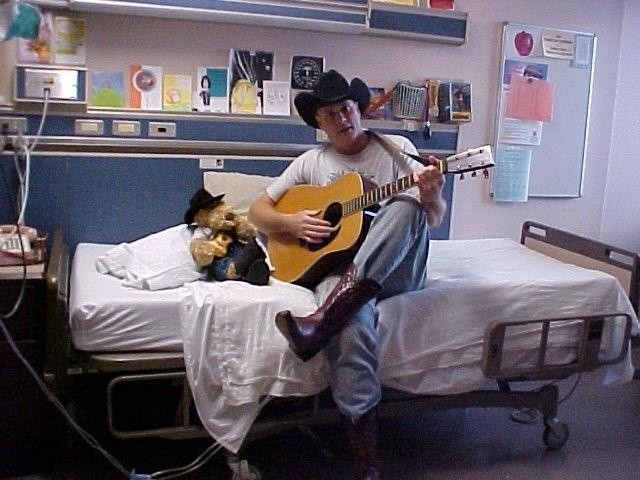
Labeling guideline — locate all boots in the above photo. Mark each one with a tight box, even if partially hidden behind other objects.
[335,414,385,479]
[275,262,383,363]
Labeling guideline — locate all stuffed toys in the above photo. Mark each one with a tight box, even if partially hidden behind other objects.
[184,189,269,285]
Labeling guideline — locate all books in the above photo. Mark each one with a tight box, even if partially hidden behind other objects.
[17,12,86,66]
[426,80,473,123]
[229,48,325,116]
[90,65,229,113]
[361,86,386,120]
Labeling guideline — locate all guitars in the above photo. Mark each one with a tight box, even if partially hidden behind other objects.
[266,145,496,293]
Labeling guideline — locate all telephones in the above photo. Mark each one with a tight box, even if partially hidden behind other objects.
[0,225,38,258]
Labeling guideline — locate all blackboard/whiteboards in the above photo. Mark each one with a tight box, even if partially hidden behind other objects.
[488,21,598,200]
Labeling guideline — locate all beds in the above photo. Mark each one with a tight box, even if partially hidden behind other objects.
[42,220,639,450]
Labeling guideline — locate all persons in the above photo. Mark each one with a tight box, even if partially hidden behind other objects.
[248,68,449,480]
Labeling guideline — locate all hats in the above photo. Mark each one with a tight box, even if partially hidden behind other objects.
[294,69,369,129]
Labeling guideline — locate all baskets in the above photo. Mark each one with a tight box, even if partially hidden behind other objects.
[393,83,427,119]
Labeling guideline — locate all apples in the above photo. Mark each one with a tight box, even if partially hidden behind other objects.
[514,31,533,56]
